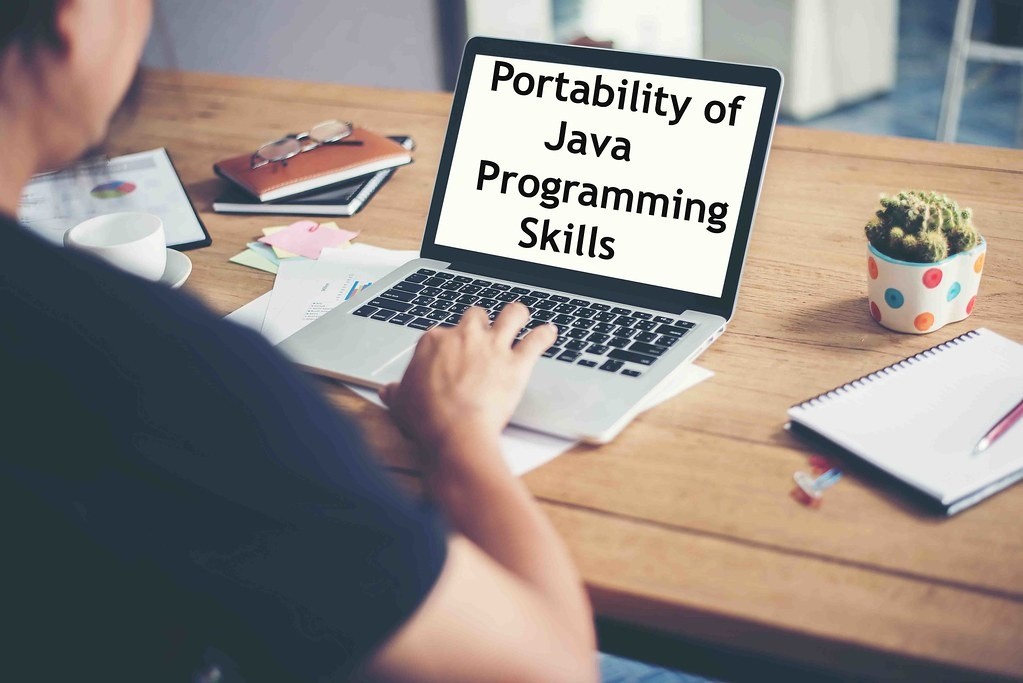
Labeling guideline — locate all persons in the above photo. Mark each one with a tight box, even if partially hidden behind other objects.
[0,0,603,683]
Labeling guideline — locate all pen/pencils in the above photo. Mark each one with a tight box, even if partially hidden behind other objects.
[970,399,1023,452]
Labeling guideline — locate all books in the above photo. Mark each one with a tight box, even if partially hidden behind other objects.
[212,120,416,202]
[781,326,1023,517]
[12,145,212,257]
[209,132,414,217]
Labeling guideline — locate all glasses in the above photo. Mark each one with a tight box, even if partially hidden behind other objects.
[248,115,364,170]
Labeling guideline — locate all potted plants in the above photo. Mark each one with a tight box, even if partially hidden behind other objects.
[860,190,990,334]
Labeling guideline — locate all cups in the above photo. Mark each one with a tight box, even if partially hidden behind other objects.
[64,210,167,284]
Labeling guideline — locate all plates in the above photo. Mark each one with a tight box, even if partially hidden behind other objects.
[161,245,193,289]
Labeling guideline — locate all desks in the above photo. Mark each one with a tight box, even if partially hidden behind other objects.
[17,69,1023,683]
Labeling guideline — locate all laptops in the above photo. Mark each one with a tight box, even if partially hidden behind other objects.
[269,38,783,448]
